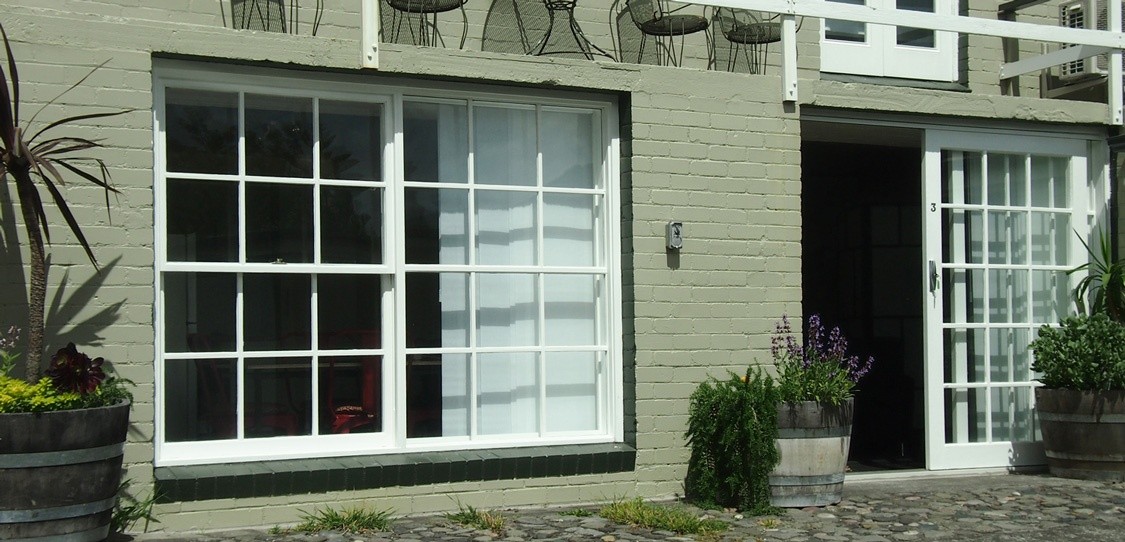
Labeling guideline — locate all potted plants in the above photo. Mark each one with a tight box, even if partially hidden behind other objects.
[0,24,151,542]
[1028,313,1125,480]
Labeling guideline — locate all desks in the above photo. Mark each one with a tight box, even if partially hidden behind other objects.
[214,360,441,435]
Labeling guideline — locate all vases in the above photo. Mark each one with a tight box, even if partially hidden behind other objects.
[770,396,857,507]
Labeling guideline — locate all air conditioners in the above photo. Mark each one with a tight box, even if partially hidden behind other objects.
[1058,0,1125,82]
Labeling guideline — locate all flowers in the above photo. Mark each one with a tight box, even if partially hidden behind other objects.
[0,325,131,415]
[684,313,875,516]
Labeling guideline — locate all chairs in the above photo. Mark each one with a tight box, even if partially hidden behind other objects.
[276,331,340,415]
[186,333,297,439]
[220,0,804,75]
[326,328,413,434]
